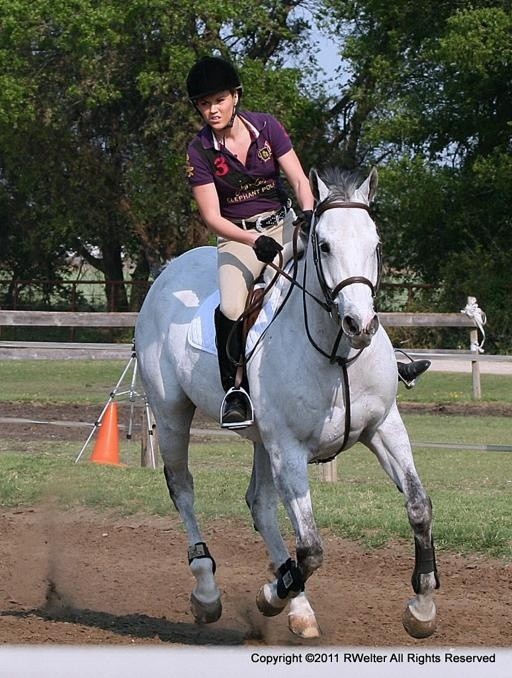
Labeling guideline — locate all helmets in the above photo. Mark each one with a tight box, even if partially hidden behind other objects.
[186,56,242,99]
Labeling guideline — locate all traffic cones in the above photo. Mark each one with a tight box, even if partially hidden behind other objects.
[90,400,129,467]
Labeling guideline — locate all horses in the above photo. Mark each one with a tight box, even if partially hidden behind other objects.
[129,163,445,644]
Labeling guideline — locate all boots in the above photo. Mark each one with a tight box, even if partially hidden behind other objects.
[214,304,252,423]
[397,360,431,381]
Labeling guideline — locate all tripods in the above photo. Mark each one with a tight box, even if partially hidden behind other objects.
[75,344,156,470]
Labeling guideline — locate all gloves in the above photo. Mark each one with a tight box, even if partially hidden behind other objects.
[292,210,313,232]
[252,235,284,263]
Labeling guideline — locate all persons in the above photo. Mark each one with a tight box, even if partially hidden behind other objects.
[183,56,431,423]
